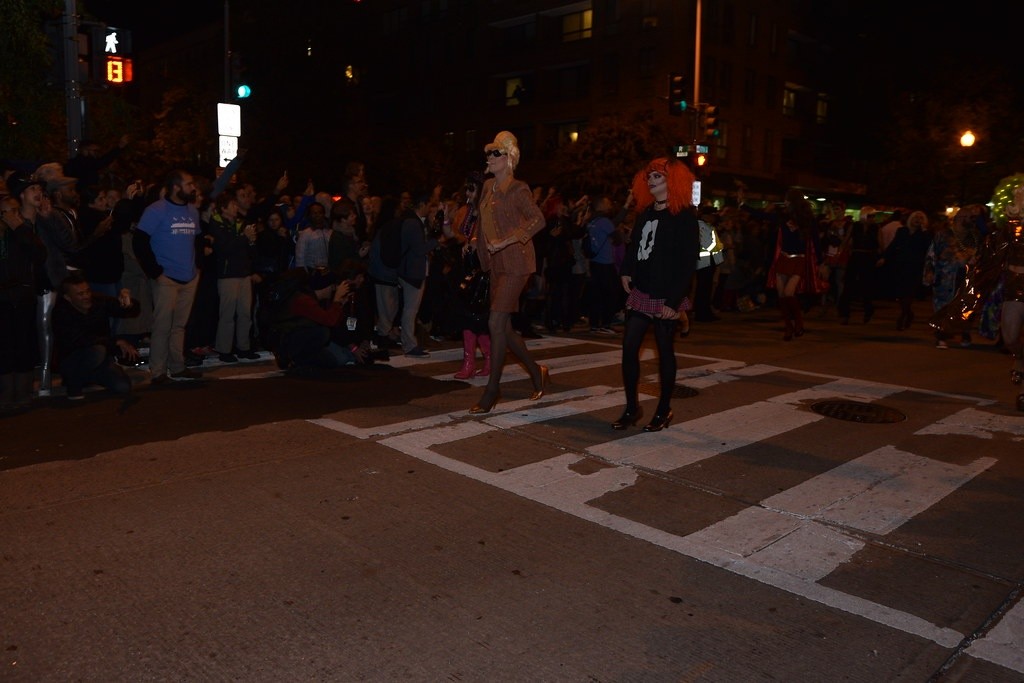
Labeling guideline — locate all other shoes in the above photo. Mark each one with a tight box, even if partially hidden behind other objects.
[903,312,914,329]
[0,347,262,418]
[368,304,718,361]
[895,319,904,331]
[961,335,972,347]
[936,339,948,349]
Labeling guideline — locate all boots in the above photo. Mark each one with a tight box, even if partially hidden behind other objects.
[454,330,476,378]
[477,335,493,377]
[788,295,804,336]
[779,304,794,339]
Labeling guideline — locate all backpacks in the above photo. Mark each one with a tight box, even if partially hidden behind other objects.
[581,217,608,258]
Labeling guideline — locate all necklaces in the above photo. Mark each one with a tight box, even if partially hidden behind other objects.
[655,199,666,205]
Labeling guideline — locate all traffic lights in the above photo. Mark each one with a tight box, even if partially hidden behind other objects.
[704,104,721,140]
[665,71,685,116]
[231,72,252,149]
[87,24,132,87]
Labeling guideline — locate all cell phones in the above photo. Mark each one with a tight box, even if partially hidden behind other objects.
[134,180,142,192]
[104,208,112,217]
[284,170,287,177]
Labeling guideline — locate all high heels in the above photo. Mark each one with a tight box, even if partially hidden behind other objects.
[529,366,551,401]
[613,404,642,430]
[643,405,673,431]
[470,385,501,414]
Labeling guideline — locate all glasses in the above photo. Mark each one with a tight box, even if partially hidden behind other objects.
[866,214,875,218]
[647,175,662,179]
[486,150,507,158]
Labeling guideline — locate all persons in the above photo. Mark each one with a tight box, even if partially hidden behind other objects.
[610,157,699,432]
[514,176,1024,409]
[0,160,635,409]
[468,131,548,414]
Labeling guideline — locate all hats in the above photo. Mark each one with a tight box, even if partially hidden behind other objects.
[49,176,79,192]
[7,176,33,198]
